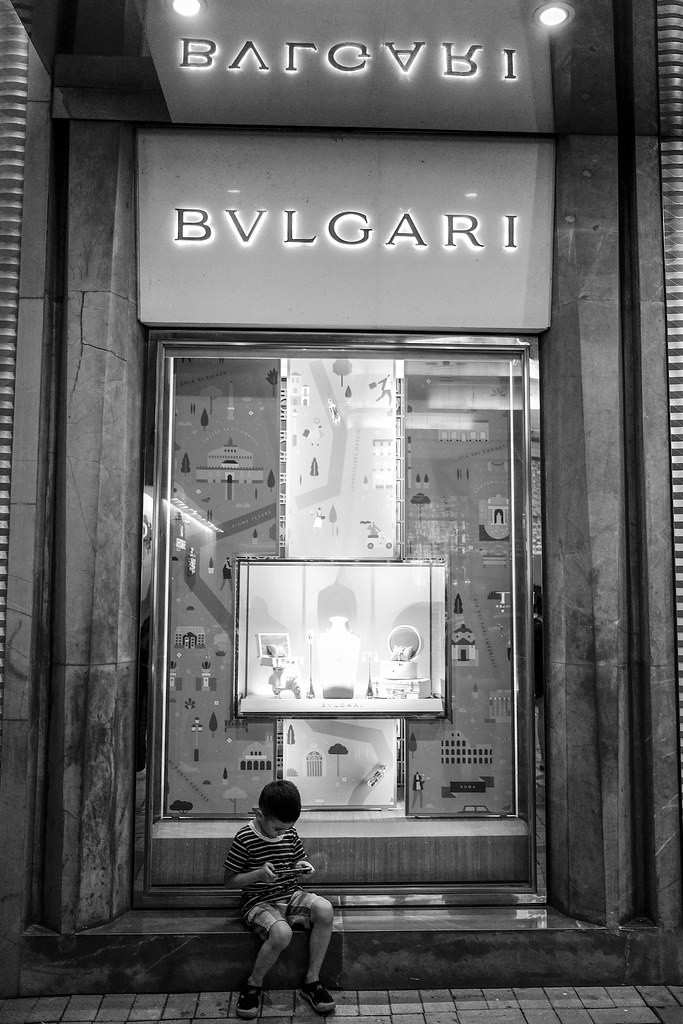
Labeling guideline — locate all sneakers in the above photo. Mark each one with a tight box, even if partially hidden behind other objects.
[235,983,263,1020]
[299,980,335,1011]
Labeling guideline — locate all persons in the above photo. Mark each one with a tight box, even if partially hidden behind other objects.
[224,779,335,1018]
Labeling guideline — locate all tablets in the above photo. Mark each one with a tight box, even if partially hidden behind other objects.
[273,865,312,874]
